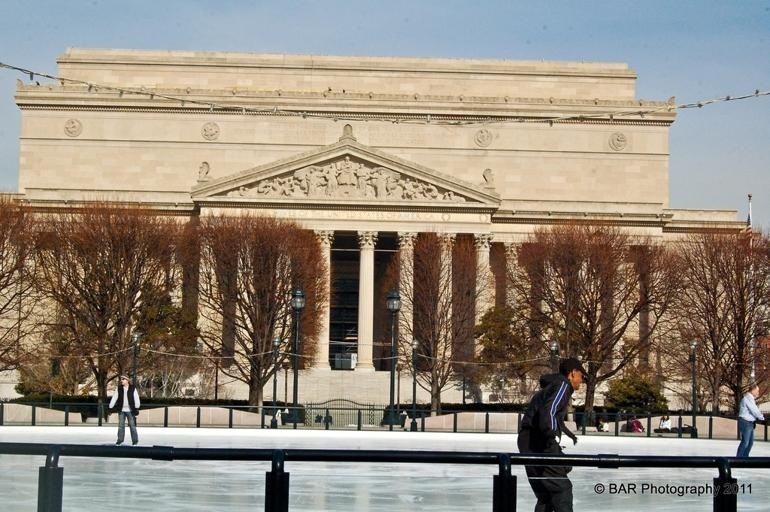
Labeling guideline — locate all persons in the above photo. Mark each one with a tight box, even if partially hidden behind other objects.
[657,414,672,437]
[108,373,142,447]
[596,414,646,435]
[256,154,440,201]
[733,383,766,457]
[515,357,586,511]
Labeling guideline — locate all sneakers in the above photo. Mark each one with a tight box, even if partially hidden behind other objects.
[115,441,140,447]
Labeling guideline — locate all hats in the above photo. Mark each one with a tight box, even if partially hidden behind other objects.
[559,358,590,377]
[120,374,130,381]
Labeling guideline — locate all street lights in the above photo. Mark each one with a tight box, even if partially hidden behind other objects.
[380,289,407,425]
[281,288,306,423]
